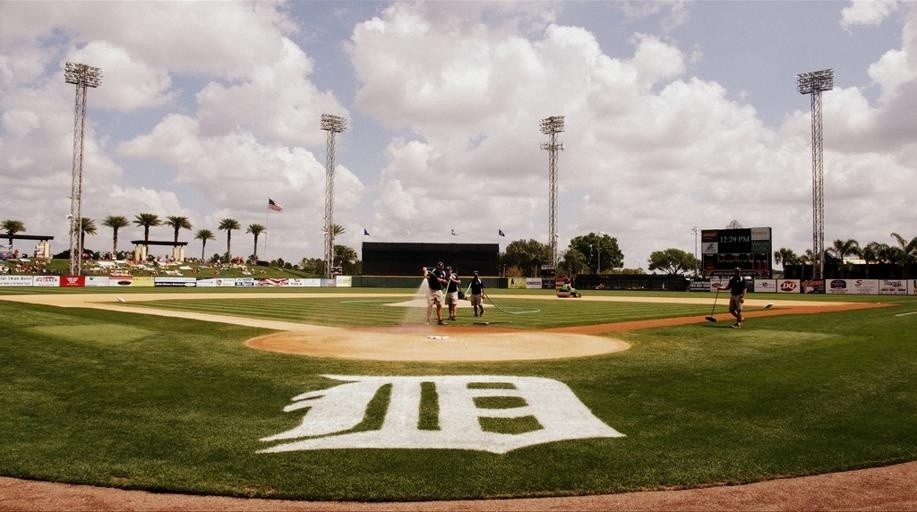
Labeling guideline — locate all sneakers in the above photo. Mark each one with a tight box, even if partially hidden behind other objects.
[438,321,448,325]
[447,316,457,320]
[731,324,742,329]
[425,320,431,326]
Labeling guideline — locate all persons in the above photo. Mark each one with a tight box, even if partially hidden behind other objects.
[0,252,266,277]
[423,262,448,326]
[559,279,576,294]
[717,267,747,329]
[467,271,485,317]
[443,266,461,321]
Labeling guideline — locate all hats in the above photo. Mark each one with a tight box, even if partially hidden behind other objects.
[446,266,452,270]
[472,271,479,275]
[436,262,445,269]
[734,267,742,272]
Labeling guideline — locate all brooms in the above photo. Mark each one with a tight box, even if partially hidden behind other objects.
[705,279,722,322]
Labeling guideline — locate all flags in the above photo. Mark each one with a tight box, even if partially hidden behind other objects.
[364,229,369,236]
[499,229,504,237]
[452,229,457,236]
[269,198,282,212]
